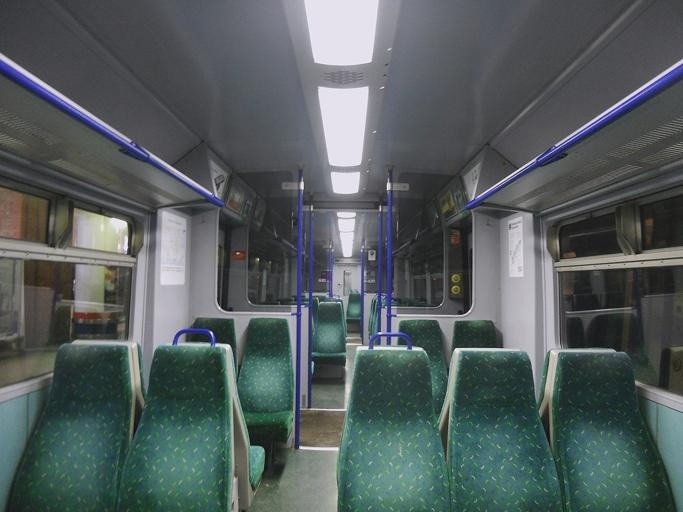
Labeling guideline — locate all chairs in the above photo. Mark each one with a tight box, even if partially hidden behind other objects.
[51,304,73,346]
[564,316,586,348]
[396,320,449,415]
[346,290,363,328]
[447,348,563,512]
[236,318,295,450]
[6,342,136,512]
[548,349,677,512]
[180,316,239,381]
[268,290,337,308]
[336,332,449,512]
[311,300,345,380]
[587,311,660,389]
[408,297,436,307]
[370,296,405,347]
[229,345,266,510]
[117,327,239,512]
[453,319,498,350]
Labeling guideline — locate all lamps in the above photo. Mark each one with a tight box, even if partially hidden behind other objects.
[338,212,356,261]
[319,83,370,167]
[331,172,362,197]
[305,1,380,66]
